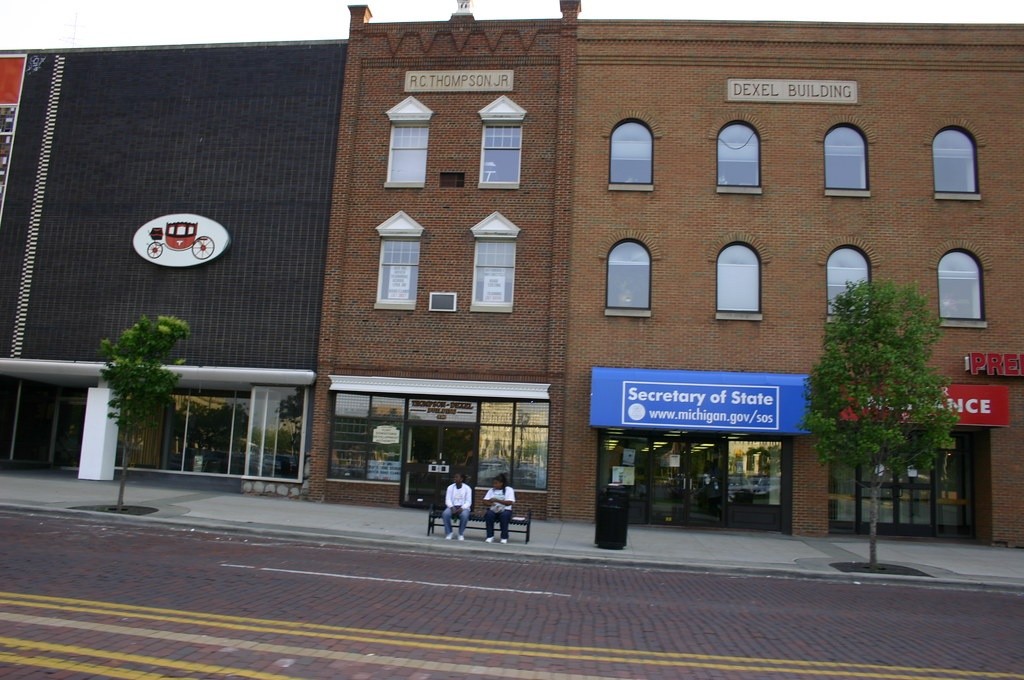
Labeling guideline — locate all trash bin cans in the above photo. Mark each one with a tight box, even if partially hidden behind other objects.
[594,484,630,550]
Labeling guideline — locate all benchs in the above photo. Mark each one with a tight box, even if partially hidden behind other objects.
[427,503,532,544]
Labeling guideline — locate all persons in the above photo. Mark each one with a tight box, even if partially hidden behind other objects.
[485,474,515,543]
[442,473,472,540]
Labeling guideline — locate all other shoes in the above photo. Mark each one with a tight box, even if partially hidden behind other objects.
[500,538,507,544]
[458,535,465,541]
[486,536,496,543]
[446,533,453,540]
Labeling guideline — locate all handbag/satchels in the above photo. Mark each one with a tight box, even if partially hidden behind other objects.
[490,503,506,513]
